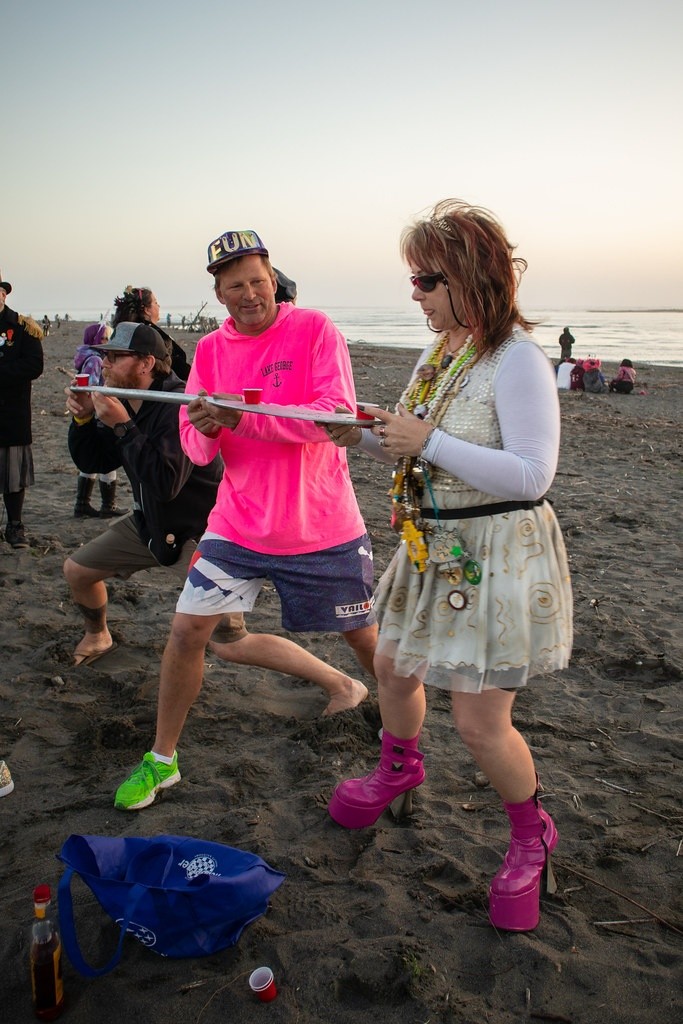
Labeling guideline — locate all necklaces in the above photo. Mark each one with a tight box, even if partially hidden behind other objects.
[392,324,486,521]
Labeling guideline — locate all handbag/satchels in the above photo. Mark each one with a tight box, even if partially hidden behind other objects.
[55,831,285,979]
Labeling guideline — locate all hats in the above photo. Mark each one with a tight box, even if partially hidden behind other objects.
[0,272,13,296]
[206,230,269,274]
[90,322,166,360]
[583,359,600,371]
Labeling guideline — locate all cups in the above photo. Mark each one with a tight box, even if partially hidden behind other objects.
[249,966,277,1002]
[356,400,378,430]
[75,373,90,386]
[243,388,263,404]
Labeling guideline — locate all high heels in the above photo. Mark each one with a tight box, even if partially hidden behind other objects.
[490,771,558,930]
[329,728,426,828]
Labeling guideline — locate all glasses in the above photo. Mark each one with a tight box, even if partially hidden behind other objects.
[410,272,444,292]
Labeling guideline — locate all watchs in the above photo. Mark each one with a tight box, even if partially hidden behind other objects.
[115,419,135,439]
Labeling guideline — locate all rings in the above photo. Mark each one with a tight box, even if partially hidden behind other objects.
[330,434,336,441]
[380,437,386,446]
[380,427,385,435]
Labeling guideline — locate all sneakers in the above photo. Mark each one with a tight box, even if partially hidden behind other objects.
[6,520,29,547]
[114,751,181,810]
[0,760,14,798]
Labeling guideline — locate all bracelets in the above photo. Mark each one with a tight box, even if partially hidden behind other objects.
[74,416,93,423]
[423,427,436,449]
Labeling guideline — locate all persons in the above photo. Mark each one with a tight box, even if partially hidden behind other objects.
[55,314,61,328]
[114,231,380,809]
[559,327,575,358]
[608,359,646,395]
[68,325,130,518]
[63,321,368,713]
[115,285,192,381]
[314,199,573,932]
[43,315,50,336]
[166,313,172,327]
[556,356,606,393]
[0,272,44,549]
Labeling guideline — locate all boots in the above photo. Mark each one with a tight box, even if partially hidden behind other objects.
[98,479,130,519]
[74,476,98,519]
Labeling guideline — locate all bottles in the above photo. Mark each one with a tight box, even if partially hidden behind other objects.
[28,884,64,1019]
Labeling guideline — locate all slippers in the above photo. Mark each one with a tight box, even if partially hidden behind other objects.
[73,641,118,668]
